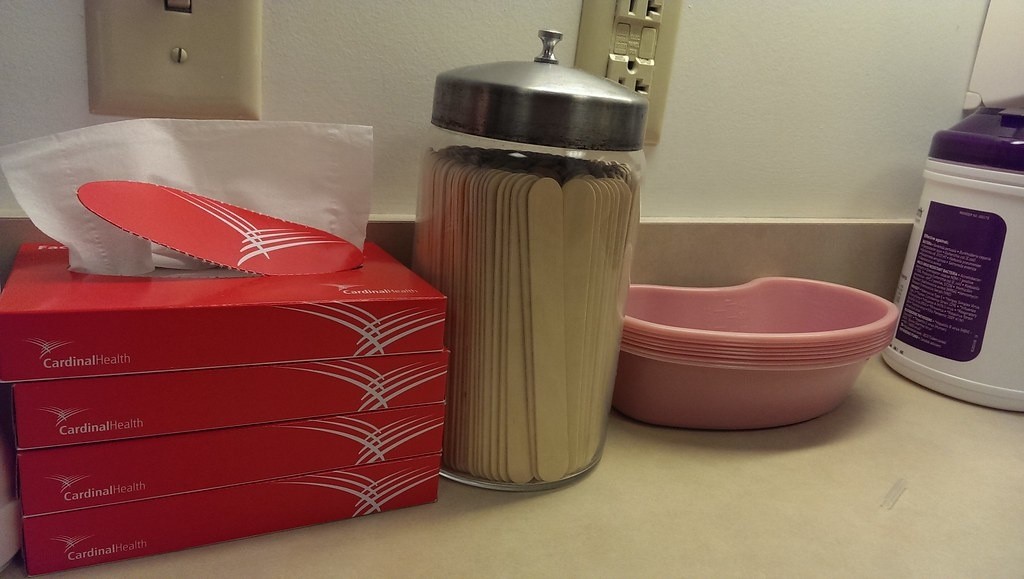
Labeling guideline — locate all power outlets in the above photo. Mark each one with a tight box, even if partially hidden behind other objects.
[574,0,680,146]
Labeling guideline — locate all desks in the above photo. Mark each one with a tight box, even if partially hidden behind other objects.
[35,357,1024,579]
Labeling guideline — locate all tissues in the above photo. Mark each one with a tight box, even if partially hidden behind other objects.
[0,117,453,579]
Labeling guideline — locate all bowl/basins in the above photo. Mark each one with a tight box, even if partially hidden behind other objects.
[612,274,903,432]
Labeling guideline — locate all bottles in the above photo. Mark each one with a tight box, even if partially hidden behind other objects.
[880,103,1024,415]
[409,26,650,491]
